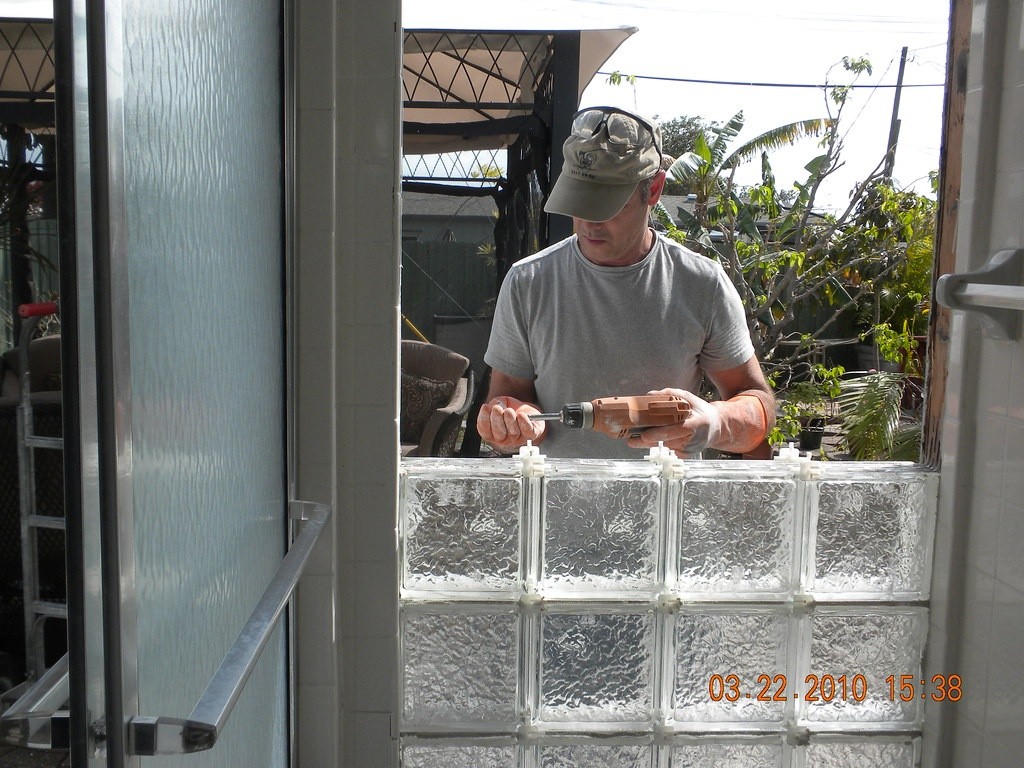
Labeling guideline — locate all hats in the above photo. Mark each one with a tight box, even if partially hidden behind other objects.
[543,106,662,223]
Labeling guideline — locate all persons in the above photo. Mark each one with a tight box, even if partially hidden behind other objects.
[476,106,778,460]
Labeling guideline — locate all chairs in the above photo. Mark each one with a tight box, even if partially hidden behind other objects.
[400,339,475,458]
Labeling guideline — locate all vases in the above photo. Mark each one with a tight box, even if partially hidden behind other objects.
[799,414,825,450]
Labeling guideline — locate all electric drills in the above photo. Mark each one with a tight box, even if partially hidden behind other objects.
[528,394,691,443]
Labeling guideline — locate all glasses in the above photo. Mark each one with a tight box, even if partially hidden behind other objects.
[570,107,662,165]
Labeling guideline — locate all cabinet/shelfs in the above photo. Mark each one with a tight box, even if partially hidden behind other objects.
[16,434,68,678]
